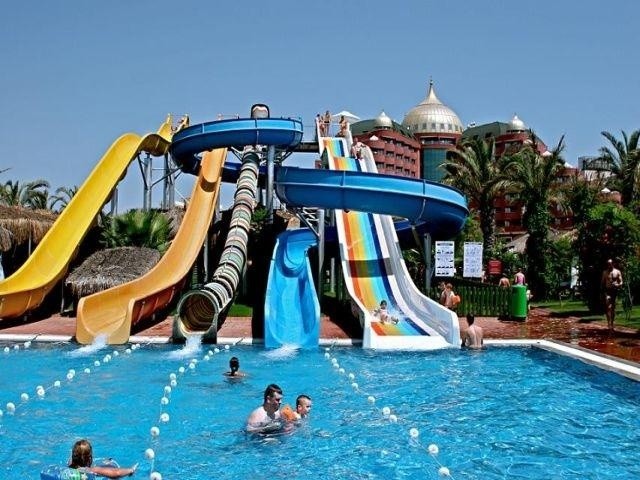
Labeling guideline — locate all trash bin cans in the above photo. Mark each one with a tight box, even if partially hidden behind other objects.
[511,285,527,320]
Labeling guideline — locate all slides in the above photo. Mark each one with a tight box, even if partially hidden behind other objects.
[165,103,271,346]
[0,112,188,323]
[75,145,227,349]
[168,116,469,351]
[309,116,463,353]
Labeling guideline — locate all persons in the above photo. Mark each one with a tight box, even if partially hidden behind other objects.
[317,110,332,136]
[374,300,399,324]
[480,267,534,317]
[350,137,367,160]
[600,258,624,333]
[461,312,484,347]
[223,357,245,376]
[339,115,347,137]
[245,383,312,438]
[437,280,458,311]
[68,440,139,479]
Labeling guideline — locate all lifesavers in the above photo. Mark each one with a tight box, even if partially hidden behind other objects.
[40,458,120,480]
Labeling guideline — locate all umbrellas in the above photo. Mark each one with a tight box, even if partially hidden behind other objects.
[331,110,361,123]
